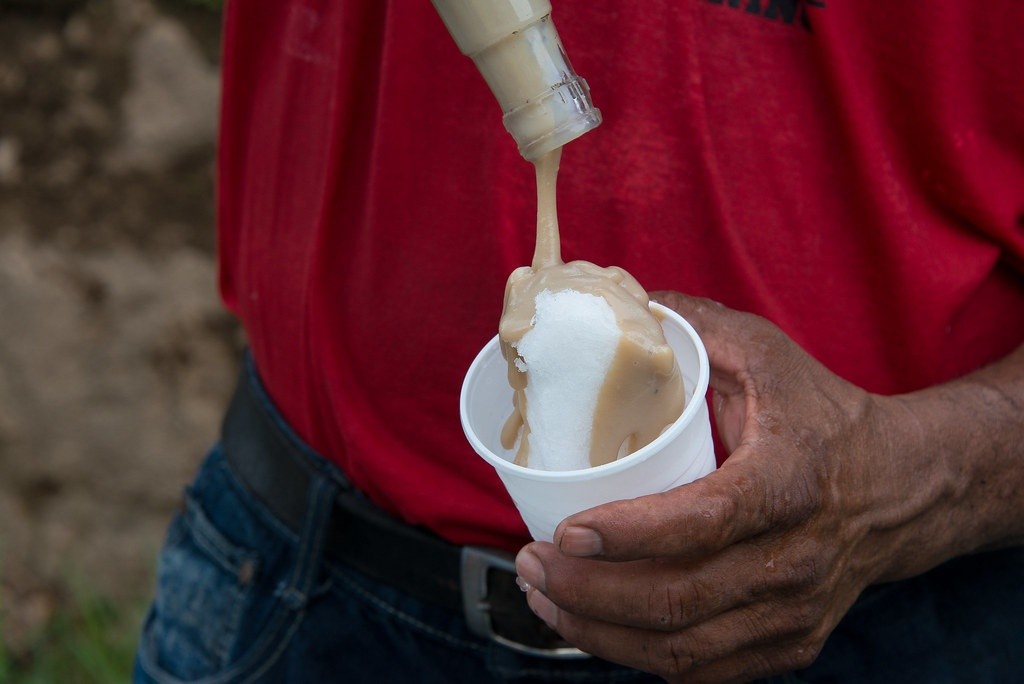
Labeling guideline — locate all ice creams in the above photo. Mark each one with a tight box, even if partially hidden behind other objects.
[498,257,687,474]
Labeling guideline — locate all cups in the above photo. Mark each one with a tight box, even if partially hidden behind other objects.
[459,299,716,546]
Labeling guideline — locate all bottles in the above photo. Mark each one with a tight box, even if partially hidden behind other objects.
[430,0,602,158]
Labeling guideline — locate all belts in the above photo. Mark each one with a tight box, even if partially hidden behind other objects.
[221,377,605,661]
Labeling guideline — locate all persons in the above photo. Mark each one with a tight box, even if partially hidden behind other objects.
[133,4,1024,684]
